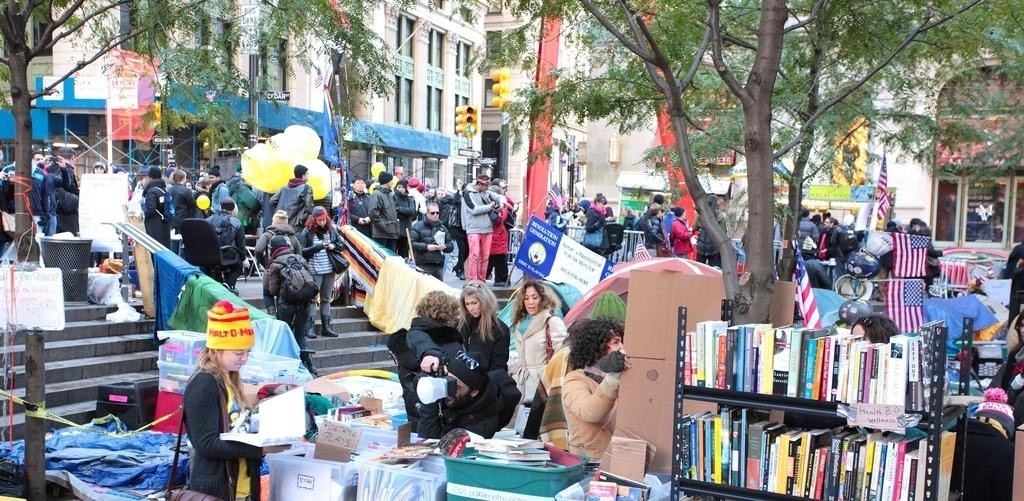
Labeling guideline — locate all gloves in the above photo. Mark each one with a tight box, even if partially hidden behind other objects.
[599,350,625,375]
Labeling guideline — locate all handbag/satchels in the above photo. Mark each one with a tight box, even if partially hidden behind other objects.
[326,247,351,274]
[583,227,603,246]
[487,202,499,221]
[164,485,227,501]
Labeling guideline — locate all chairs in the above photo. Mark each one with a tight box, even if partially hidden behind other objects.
[241,235,265,281]
[179,217,246,298]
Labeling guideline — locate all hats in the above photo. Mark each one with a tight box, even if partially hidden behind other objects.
[448,346,489,390]
[589,289,627,325]
[205,299,256,350]
[407,177,424,192]
[195,165,220,192]
[58,148,75,156]
[379,172,394,185]
[313,205,328,220]
[237,163,242,172]
[654,194,685,218]
[220,199,236,211]
[477,174,491,186]
[268,236,291,249]
[270,210,289,225]
[396,180,407,190]
[294,165,308,178]
[94,161,178,179]
[973,386,1016,439]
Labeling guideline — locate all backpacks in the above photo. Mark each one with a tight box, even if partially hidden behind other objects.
[504,206,518,230]
[697,225,719,256]
[268,253,320,302]
[266,228,294,259]
[218,216,238,262]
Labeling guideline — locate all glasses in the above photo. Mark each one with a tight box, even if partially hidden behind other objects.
[429,211,440,215]
[454,349,484,375]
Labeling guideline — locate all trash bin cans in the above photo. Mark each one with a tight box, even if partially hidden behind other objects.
[41,238,93,306]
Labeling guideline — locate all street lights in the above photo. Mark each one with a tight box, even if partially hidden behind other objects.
[152,134,173,144]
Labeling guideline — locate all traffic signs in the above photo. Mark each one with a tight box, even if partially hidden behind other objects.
[459,148,483,159]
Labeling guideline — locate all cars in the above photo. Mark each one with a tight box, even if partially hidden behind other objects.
[924,247,1009,300]
[958,208,999,240]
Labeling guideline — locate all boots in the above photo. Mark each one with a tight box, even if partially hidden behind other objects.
[305,318,318,338]
[321,317,340,337]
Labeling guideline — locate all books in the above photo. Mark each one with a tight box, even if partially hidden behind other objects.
[584,469,652,501]
[678,319,959,501]
[463,434,554,469]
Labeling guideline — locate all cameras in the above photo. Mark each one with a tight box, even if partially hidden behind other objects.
[415,364,457,405]
[49,157,58,163]
[5,173,14,179]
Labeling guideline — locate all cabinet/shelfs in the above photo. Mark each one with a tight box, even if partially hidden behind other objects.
[668,302,979,501]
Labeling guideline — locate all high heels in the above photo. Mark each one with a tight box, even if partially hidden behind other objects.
[452,264,467,280]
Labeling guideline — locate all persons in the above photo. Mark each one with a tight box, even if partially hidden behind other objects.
[180,297,285,501]
[949,386,1016,501]
[559,317,631,471]
[1,143,1023,440]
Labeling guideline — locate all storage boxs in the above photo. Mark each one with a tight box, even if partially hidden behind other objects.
[150,390,187,437]
[153,360,199,398]
[237,350,302,387]
[157,329,206,365]
[94,375,144,431]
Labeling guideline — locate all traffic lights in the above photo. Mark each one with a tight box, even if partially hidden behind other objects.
[489,69,500,109]
[465,106,475,134]
[454,107,462,135]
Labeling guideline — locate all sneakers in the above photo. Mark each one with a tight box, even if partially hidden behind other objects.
[493,281,511,287]
